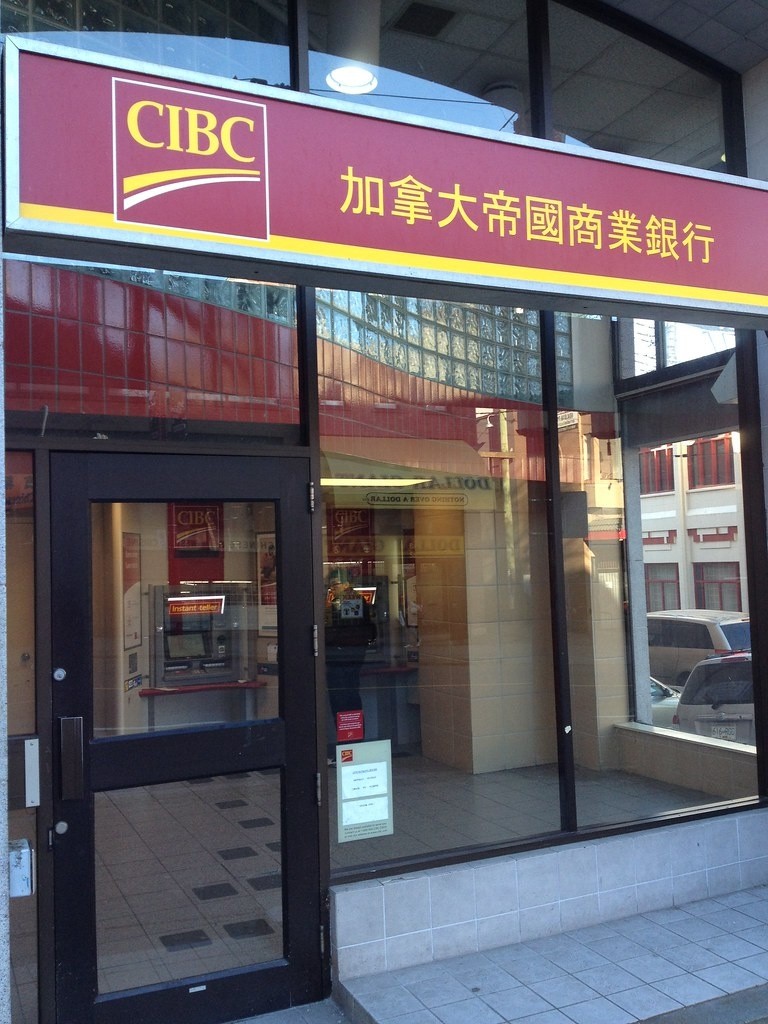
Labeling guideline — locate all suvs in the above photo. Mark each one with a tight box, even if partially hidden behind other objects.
[672,649,756,748]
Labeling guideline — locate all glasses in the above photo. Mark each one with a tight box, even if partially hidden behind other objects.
[329,583,342,587]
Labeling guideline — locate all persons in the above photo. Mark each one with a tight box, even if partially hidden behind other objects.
[261,544,275,581]
[325,568,373,769]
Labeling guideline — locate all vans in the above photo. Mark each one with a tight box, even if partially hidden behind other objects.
[647,610,751,686]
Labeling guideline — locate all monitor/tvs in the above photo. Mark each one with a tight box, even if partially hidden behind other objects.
[164,630,212,661]
